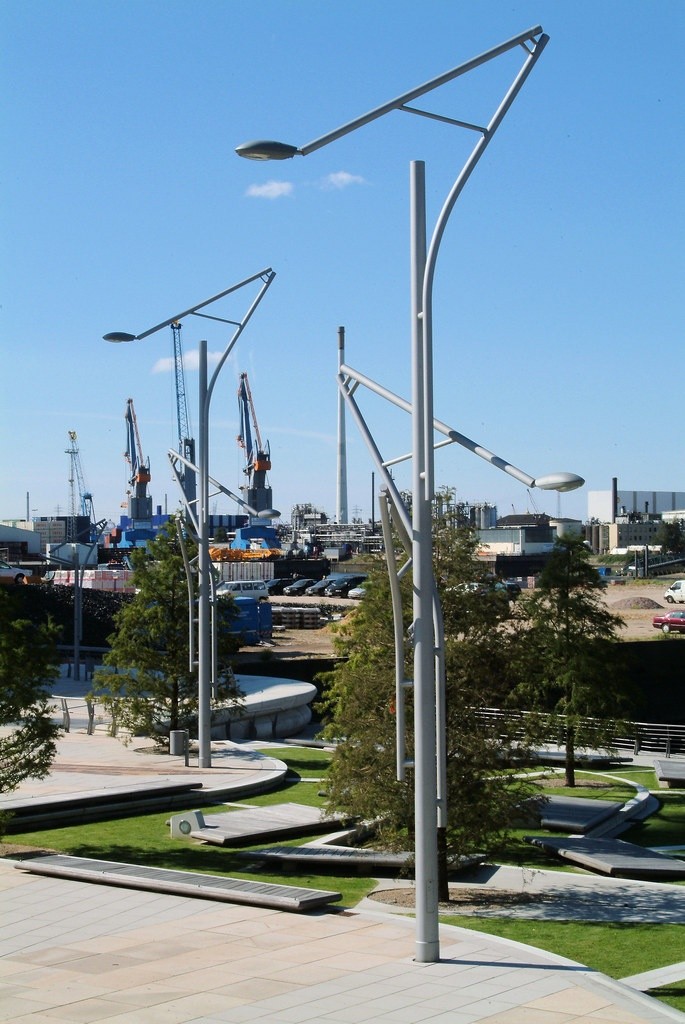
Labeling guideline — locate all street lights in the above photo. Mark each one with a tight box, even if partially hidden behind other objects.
[101,265,277,768]
[37,518,109,682]
[233,22,589,965]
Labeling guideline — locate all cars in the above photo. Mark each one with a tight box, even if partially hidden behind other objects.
[283,579,318,596]
[257,578,299,596]
[347,587,366,600]
[0,561,33,584]
[444,581,487,596]
[652,610,685,635]
[305,579,336,596]
[324,573,368,598]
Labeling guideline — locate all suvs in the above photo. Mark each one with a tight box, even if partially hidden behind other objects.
[495,582,521,598]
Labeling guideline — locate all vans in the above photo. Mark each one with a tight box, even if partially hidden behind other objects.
[663,579,685,604]
[215,581,269,603]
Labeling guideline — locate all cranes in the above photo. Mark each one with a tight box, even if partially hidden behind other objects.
[63,429,98,543]
[232,371,279,552]
[121,396,160,549]
[167,319,198,545]
